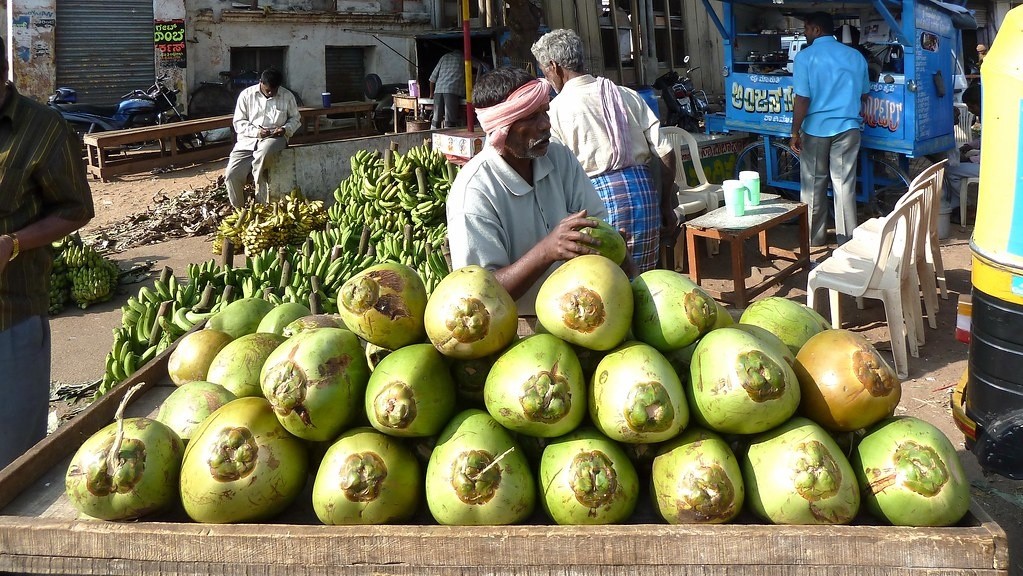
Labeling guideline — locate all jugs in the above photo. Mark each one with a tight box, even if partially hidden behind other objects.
[408,79,417,97]
[739,171,759,206]
[721,180,751,217]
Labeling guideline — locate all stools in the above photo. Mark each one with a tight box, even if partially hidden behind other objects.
[960,178,979,227]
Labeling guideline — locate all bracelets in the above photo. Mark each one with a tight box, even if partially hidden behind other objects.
[3,233,19,261]
[790,134,799,138]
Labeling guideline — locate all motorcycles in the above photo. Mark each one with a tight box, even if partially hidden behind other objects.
[44,73,205,162]
[650,56,711,133]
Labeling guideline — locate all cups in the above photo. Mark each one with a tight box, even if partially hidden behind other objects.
[322,92,331,109]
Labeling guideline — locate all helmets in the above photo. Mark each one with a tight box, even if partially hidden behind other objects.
[50,87,77,103]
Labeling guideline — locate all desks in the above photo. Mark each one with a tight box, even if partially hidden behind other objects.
[685,193,810,308]
[391,90,418,134]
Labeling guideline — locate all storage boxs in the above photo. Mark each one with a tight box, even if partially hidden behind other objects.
[2,315,1010,575]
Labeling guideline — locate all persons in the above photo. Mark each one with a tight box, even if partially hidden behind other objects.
[428,46,466,130]
[225,67,301,209]
[446,66,631,318]
[531,28,677,280]
[0,37,95,471]
[946,85,982,225]
[790,10,871,247]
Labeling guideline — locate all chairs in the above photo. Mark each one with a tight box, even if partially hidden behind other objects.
[954,103,974,148]
[808,158,948,379]
[656,126,728,259]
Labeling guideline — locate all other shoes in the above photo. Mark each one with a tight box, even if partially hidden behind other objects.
[950,216,975,225]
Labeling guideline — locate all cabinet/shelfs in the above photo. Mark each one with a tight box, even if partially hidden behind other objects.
[733,30,795,64]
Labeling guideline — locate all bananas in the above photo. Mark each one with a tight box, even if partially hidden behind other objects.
[47,142,459,402]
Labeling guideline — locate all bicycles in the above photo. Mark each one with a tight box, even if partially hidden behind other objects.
[188,68,304,120]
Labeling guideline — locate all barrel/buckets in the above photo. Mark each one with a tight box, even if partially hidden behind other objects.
[959,234,1023,481]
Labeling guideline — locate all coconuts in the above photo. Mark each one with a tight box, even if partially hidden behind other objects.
[565,216,627,267]
[66,256,972,528]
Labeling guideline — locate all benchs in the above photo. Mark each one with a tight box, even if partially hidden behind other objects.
[84,101,372,182]
